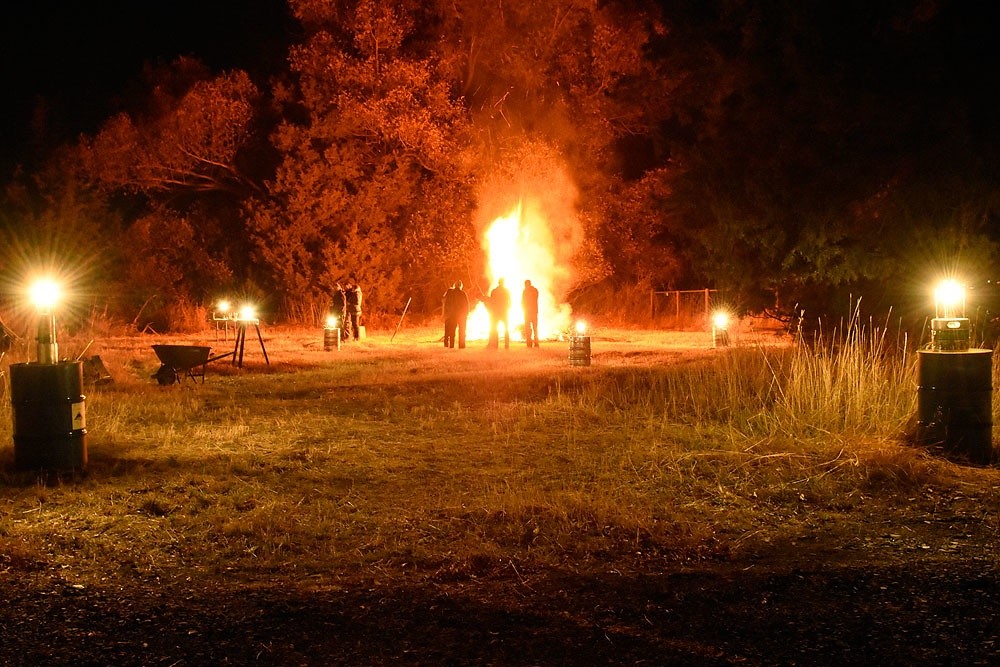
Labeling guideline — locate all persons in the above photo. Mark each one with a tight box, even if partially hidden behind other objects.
[487,278,510,349]
[442,280,470,349]
[332,280,362,343]
[522,280,539,348]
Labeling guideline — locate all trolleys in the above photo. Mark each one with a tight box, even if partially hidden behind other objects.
[151,345,234,385]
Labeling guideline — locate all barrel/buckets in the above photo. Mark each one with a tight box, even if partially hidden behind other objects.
[570,336,590,368]
[931,319,969,350]
[916,348,993,467]
[9,360,88,474]
[323,328,340,351]
[713,326,726,347]
